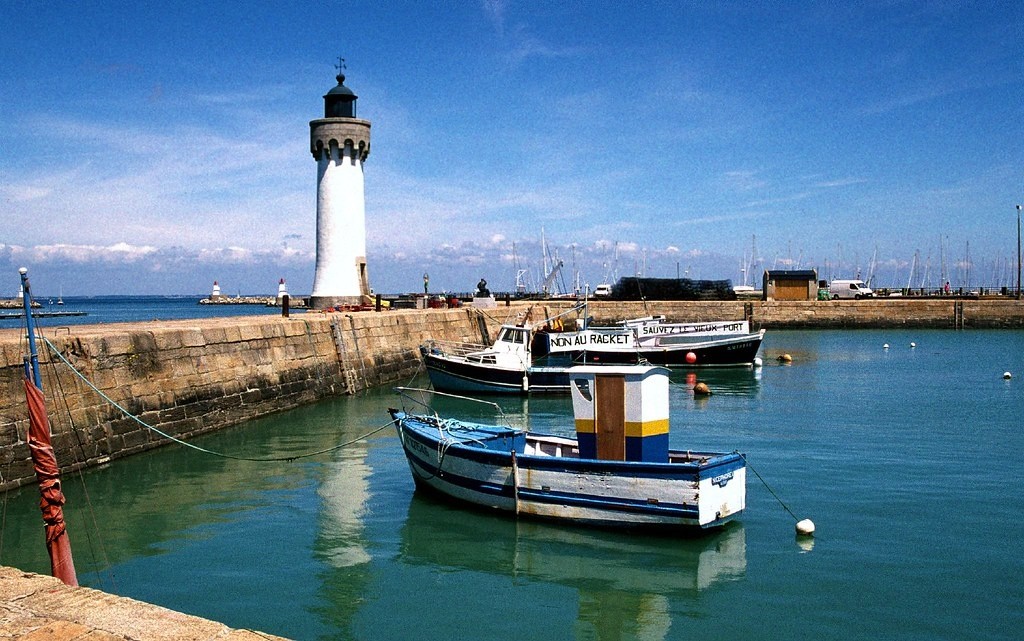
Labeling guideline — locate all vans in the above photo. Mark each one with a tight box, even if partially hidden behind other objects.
[830,279,873,300]
[595,286,611,296]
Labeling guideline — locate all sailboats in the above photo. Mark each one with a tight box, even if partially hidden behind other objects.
[56,284,66,304]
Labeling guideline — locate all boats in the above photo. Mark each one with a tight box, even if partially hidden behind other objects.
[534,232,767,368]
[420,228,660,395]
[386,358,747,533]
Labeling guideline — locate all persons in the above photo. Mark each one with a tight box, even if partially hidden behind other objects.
[945,282,949,295]
[477,279,490,297]
